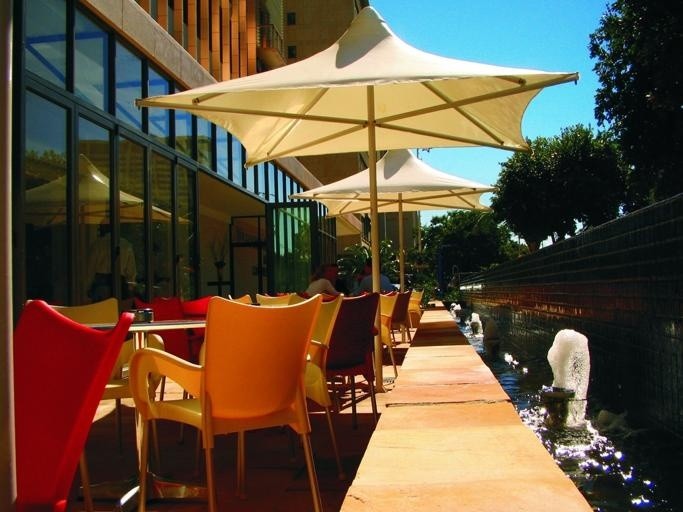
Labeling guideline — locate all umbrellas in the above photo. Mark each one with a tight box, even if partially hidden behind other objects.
[132,4,580,396]
[286,151,499,343]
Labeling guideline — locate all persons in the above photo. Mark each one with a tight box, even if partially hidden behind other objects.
[304,264,341,301]
[347,258,392,296]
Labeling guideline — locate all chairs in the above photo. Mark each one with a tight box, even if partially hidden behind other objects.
[15,287,425,512]
